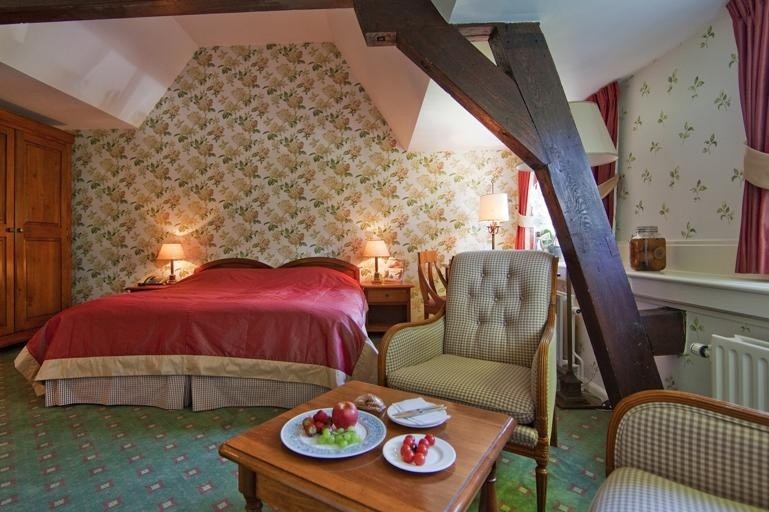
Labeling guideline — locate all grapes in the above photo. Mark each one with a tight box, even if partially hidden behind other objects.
[318,425,360,447]
[401,433,434,465]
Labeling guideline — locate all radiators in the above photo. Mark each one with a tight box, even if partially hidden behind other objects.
[556,290,579,369]
[689,332,769,415]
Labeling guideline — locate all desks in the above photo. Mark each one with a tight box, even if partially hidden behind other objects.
[218,378,518,511]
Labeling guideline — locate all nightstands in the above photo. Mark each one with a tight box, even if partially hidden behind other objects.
[358,279,415,333]
[125,283,173,291]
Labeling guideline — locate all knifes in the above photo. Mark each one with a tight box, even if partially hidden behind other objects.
[394,404,448,418]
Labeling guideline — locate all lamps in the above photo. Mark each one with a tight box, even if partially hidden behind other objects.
[363,240,391,282]
[157,241,186,284]
[515,101,620,173]
[478,192,510,248]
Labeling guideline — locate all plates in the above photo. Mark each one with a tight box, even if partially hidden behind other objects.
[386,398,450,430]
[378,434,458,473]
[279,407,386,461]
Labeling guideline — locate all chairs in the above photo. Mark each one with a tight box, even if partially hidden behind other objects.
[417,250,450,319]
[588,386,769,511]
[377,248,561,512]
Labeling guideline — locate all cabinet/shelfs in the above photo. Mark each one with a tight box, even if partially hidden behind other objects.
[0,108,76,347]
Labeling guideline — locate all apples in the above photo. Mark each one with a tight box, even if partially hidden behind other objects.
[332,401,358,428]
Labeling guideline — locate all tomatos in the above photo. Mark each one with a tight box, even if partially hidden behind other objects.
[303,410,333,437]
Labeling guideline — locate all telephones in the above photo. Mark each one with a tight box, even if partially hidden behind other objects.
[144,275,168,284]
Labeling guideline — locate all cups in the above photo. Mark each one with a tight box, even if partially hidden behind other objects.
[552,246,561,258]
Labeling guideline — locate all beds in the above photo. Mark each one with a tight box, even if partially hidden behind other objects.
[19,256,368,410]
